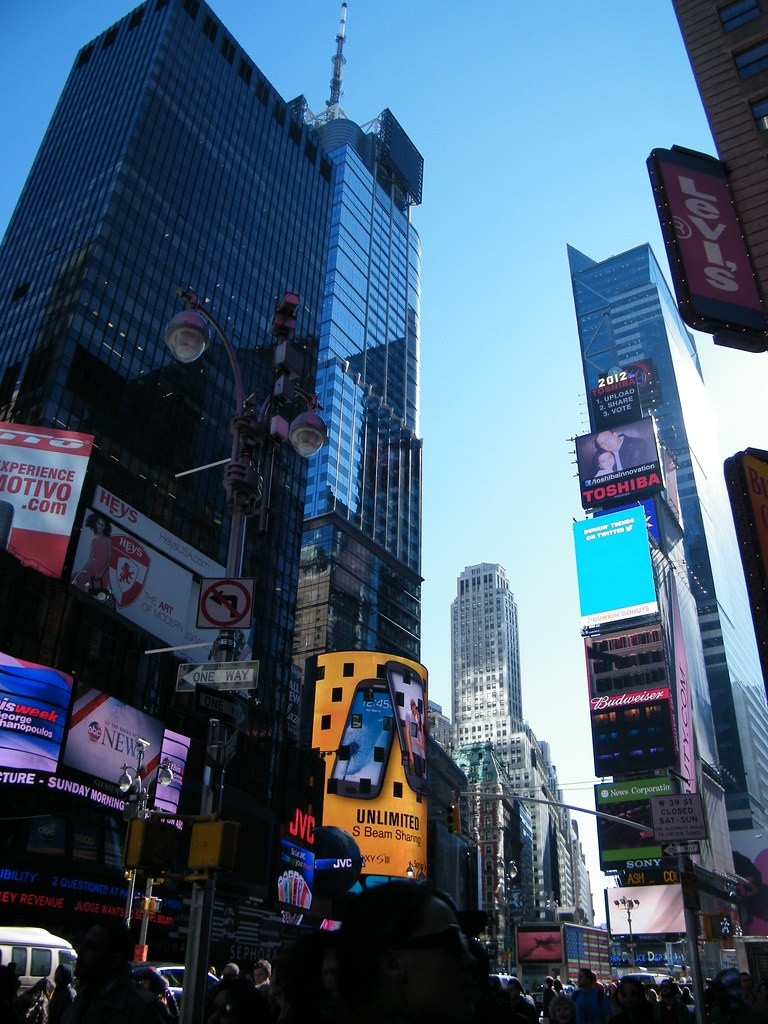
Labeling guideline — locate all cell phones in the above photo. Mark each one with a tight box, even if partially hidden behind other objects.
[331,677,396,800]
[384,660,428,796]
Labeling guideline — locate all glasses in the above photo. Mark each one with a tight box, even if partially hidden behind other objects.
[618,990,639,998]
[253,963,266,970]
[389,923,470,967]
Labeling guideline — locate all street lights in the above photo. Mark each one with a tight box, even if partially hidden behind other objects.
[116,737,173,936]
[161,285,327,1011]
[615,898,640,970]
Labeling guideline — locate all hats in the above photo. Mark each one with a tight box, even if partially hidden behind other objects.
[544,977,553,986]
[554,980,563,990]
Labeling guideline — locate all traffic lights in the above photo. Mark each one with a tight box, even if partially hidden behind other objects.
[703,911,738,941]
[447,804,462,835]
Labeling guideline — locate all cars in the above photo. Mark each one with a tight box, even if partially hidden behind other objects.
[106,962,220,1014]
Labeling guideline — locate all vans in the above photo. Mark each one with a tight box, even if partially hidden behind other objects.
[0,926,80,1000]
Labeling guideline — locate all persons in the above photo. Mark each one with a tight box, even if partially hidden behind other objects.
[70,514,113,594]
[405,699,426,780]
[0,928,768,1024]
[591,430,650,478]
[336,881,478,1024]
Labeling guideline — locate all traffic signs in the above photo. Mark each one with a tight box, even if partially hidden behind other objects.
[660,839,703,857]
